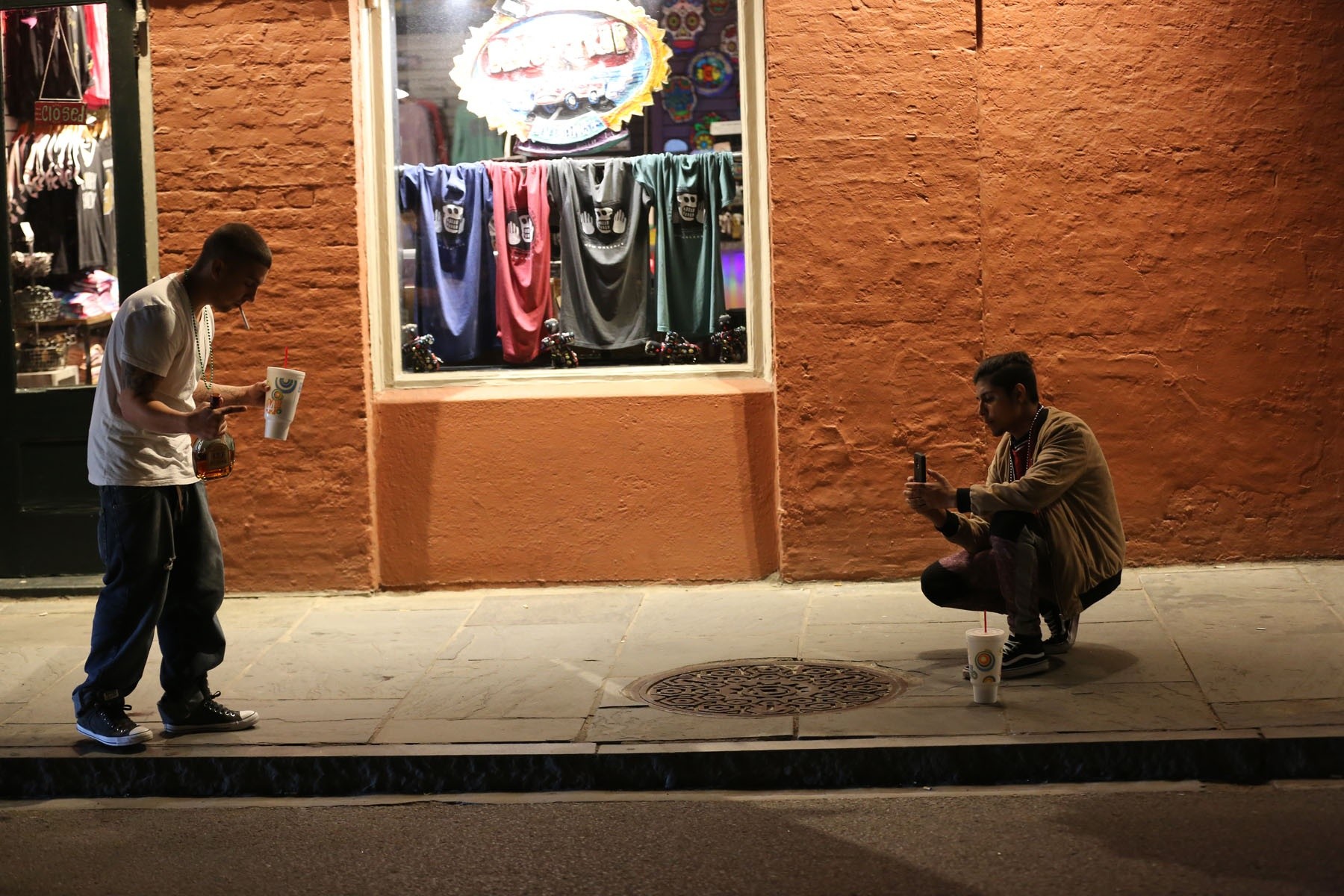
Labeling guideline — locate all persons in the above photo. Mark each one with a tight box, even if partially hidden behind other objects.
[70,224,300,745]
[902,351,1125,679]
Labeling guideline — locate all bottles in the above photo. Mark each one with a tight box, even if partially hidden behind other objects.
[193,393,236,480]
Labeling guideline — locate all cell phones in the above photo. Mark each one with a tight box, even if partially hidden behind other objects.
[914,452,927,484]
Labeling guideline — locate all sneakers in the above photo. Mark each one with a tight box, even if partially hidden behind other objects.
[163,691,259,733]
[1041,604,1080,653]
[962,634,1050,680]
[75,698,154,747]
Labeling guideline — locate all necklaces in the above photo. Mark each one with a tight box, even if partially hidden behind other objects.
[184,268,214,389]
[1010,405,1044,483]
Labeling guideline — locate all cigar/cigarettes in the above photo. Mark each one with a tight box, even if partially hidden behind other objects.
[238,305,250,330]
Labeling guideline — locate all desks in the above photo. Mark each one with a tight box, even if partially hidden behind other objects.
[16,311,113,385]
[17,365,79,388]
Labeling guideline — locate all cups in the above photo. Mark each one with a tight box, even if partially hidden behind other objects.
[967,628,1005,705]
[265,366,306,440]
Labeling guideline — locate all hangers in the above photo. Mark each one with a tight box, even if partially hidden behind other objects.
[10,108,110,153]
